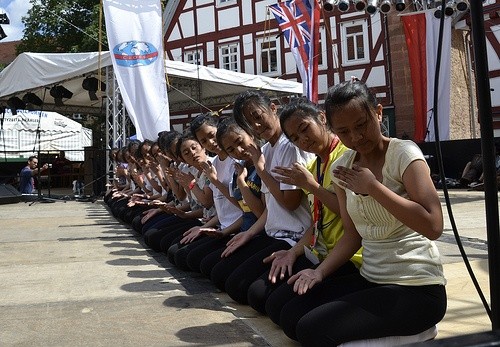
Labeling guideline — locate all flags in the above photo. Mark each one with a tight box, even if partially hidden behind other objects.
[102,0,170,142]
[397,8,452,142]
[265,0,321,105]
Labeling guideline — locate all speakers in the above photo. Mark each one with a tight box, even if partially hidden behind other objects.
[0,183,22,204]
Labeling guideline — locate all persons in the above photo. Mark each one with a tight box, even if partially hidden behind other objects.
[103,77,448,347]
[18,156,49,195]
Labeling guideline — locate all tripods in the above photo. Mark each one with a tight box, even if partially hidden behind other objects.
[24,88,66,206]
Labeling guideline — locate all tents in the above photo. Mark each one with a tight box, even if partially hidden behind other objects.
[0,52,304,116]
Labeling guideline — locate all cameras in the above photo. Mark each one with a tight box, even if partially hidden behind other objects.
[46,163,52,168]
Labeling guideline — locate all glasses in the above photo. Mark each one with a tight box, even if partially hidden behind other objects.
[33,161,38,165]
